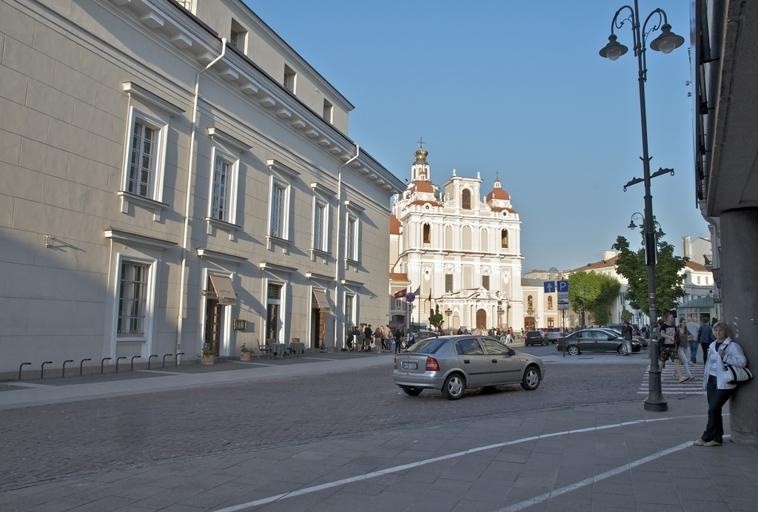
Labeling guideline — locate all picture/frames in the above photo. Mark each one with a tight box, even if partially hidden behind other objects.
[234,320,246,330]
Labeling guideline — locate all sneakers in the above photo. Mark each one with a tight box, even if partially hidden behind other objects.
[673,375,694,383]
[695,439,721,447]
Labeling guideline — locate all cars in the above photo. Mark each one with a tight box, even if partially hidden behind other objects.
[392,333,547,402]
[524,323,649,355]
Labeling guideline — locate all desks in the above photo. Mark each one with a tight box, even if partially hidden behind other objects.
[290,343,305,354]
[271,344,287,358]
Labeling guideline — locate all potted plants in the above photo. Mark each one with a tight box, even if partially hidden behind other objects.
[201,344,216,366]
[240,343,251,362]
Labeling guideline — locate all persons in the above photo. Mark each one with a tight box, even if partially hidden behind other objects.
[674,317,696,383]
[621,320,633,357]
[345,323,403,356]
[536,327,564,331]
[686,312,721,365]
[659,312,689,384]
[694,321,754,447]
[644,323,650,339]
[456,325,526,344]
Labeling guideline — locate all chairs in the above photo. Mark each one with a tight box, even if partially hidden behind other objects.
[268,339,275,345]
[257,339,272,358]
[292,338,300,342]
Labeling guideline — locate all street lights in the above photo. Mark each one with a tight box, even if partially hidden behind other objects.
[599,1,692,415]
[624,206,672,260]
[497,307,506,331]
[444,307,454,335]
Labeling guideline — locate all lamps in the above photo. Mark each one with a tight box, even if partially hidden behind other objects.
[44,234,55,247]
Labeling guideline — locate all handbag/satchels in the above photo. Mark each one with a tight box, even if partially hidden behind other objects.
[723,363,753,383]
